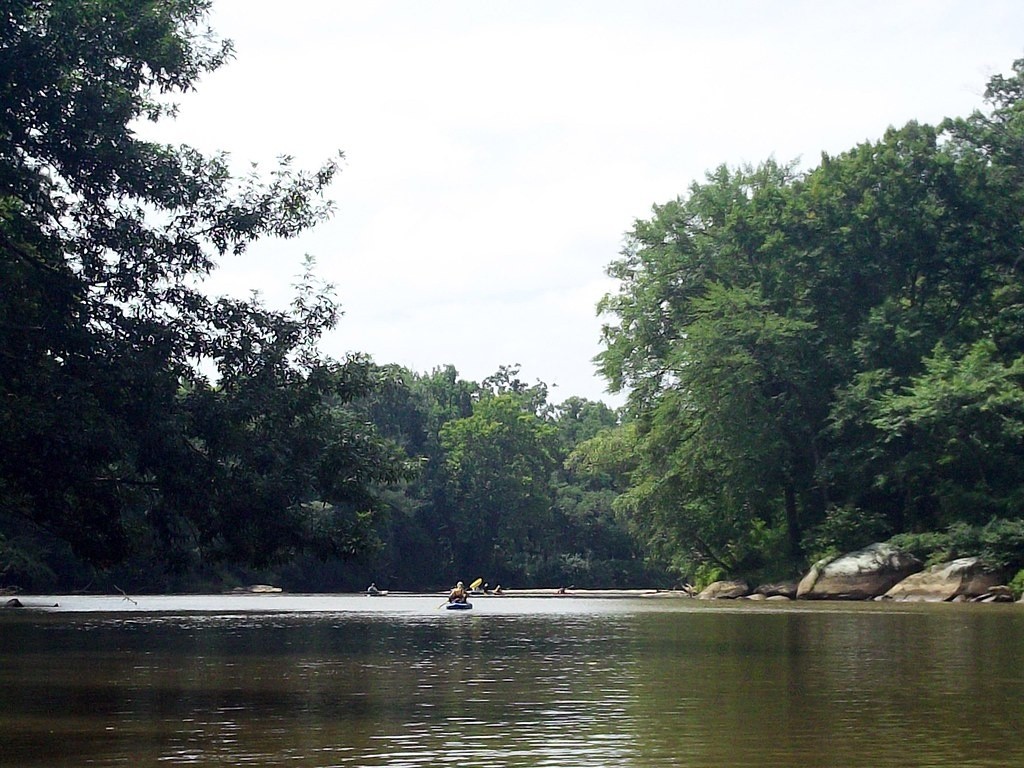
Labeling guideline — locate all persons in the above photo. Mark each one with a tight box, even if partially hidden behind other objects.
[495,585,502,594]
[482,581,489,593]
[368,583,378,592]
[448,581,468,603]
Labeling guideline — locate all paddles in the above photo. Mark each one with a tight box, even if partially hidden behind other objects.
[436,577,484,610]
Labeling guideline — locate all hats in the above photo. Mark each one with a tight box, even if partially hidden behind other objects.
[457,581,463,587]
[372,583,375,585]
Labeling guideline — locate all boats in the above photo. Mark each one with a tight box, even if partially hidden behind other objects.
[445,602,473,610]
[250,584,282,594]
[364,589,389,596]
